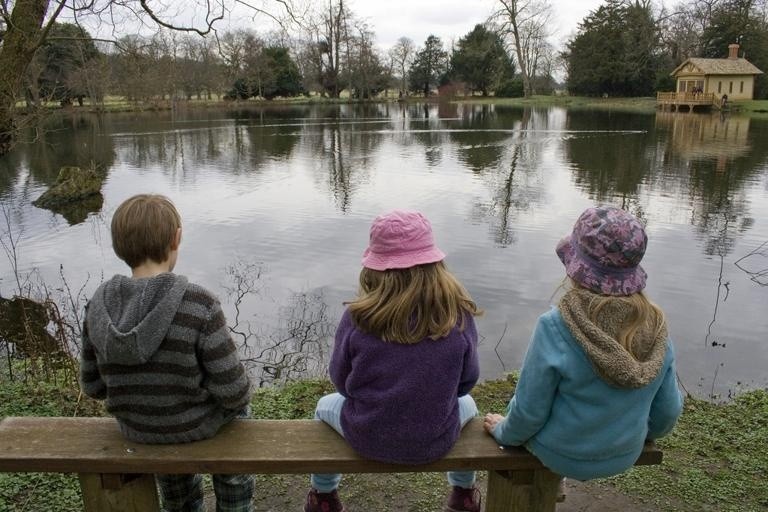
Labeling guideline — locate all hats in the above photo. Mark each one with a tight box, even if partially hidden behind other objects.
[362,210,444,271]
[555,203,648,295]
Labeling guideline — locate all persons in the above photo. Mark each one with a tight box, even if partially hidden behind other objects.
[484,206,681,501]
[80,192,255,512]
[304,210,482,511]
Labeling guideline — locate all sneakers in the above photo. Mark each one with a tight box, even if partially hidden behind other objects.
[442,485,482,511]
[304,490,346,512]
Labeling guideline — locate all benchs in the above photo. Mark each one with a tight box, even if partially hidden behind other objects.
[0,416,673,512]
